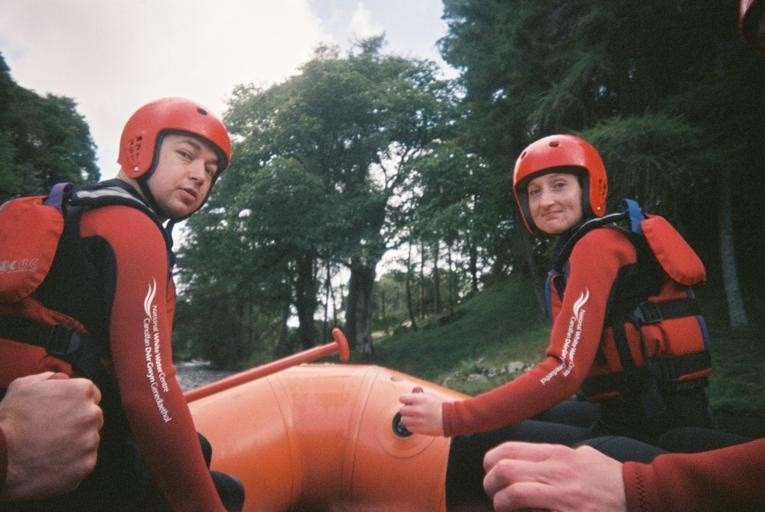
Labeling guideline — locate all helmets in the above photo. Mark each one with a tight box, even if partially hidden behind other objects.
[512,133,608,235]
[117,97,232,179]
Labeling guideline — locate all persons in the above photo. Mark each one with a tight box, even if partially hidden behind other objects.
[1,97,244,511]
[399,133,713,512]
[481,435,764,512]
[0,371,105,512]
